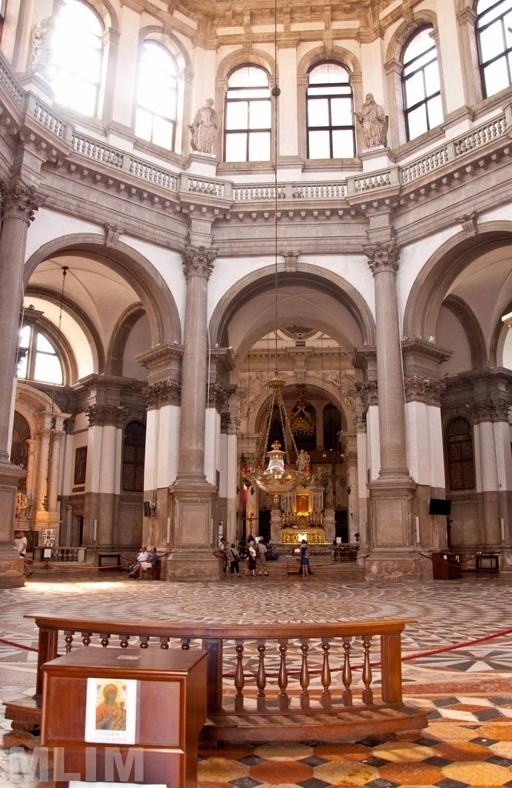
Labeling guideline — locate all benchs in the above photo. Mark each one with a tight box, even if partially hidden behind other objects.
[128,560,162,579]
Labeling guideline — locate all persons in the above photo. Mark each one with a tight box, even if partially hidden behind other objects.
[15,531,33,576]
[127,546,158,579]
[188,97,219,151]
[95,684,127,729]
[296,449,308,471]
[300,539,312,577]
[304,451,310,464]
[353,92,386,147]
[218,534,270,576]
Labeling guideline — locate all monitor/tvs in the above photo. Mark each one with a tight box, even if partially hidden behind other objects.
[429,499,451,515]
[143,502,150,517]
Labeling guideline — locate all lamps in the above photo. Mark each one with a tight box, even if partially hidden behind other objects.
[233,0,315,495]
